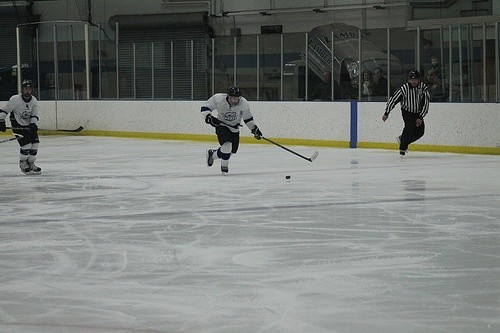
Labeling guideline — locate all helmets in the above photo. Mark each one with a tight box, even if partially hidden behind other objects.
[408,70,420,80]
[226,86,241,96]
[21,79,33,89]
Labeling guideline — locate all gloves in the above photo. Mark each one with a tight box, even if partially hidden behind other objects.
[0,119,6,132]
[251,124,262,140]
[28,123,37,139]
[205,114,222,127]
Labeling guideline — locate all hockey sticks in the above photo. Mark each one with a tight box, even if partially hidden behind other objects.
[1,133,22,144]
[252,133,319,162]
[6,119,91,133]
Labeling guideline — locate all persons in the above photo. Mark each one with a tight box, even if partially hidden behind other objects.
[0,80,42,175]
[361,68,397,102]
[304,71,342,101]
[425,69,449,102]
[381,70,431,158]
[200,85,263,174]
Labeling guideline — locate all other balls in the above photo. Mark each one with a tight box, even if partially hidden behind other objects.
[285,175,291,180]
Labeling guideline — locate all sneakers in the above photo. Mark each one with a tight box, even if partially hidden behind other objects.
[221,165,229,176]
[396,135,402,143]
[19,155,42,176]
[400,150,405,158]
[206,149,216,168]
